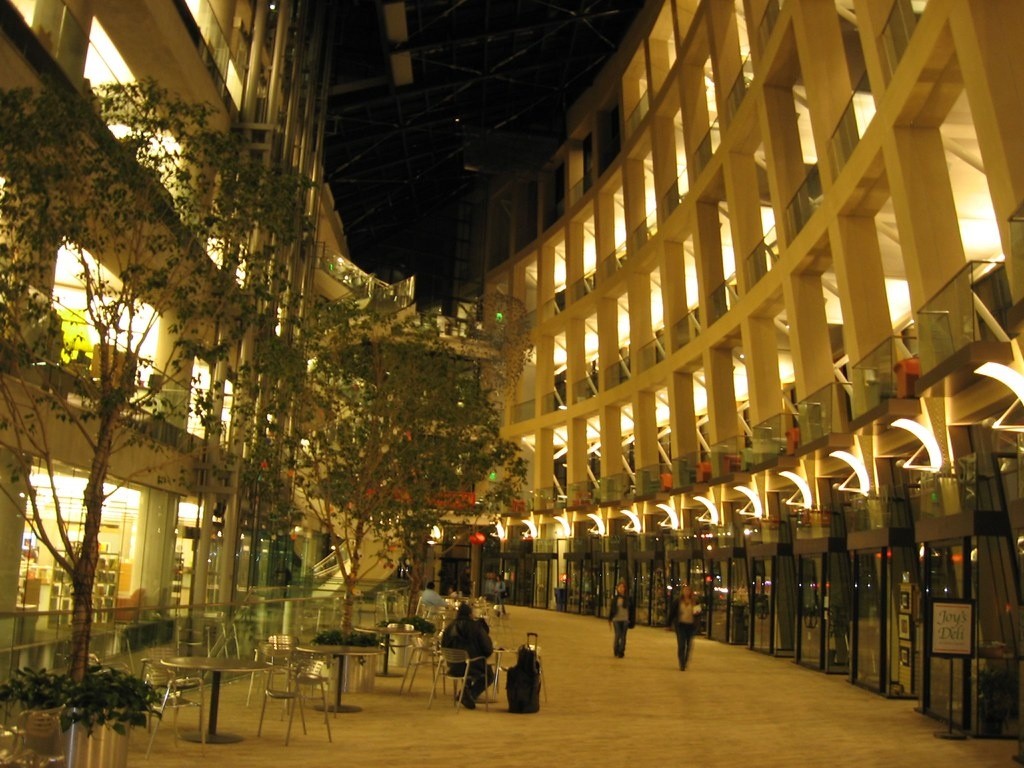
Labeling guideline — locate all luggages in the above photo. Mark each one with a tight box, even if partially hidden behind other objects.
[507,632,540,712]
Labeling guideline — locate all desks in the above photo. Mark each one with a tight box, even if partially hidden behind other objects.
[160,657,272,744]
[353,626,422,678]
[295,644,386,713]
[487,648,519,704]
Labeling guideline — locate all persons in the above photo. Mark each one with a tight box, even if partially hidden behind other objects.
[667,586,699,671]
[423,583,446,608]
[442,604,494,709]
[485,573,505,615]
[608,581,630,658]
[448,586,458,598]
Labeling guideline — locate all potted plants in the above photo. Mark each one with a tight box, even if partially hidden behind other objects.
[975,669,1016,736]
[0,665,66,755]
[58,661,163,768]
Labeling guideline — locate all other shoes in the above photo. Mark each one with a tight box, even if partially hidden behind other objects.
[680,665,685,671]
[619,651,624,659]
[455,692,476,709]
[614,647,619,655]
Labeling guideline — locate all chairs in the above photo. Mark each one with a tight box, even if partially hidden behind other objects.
[0,592,550,768]
